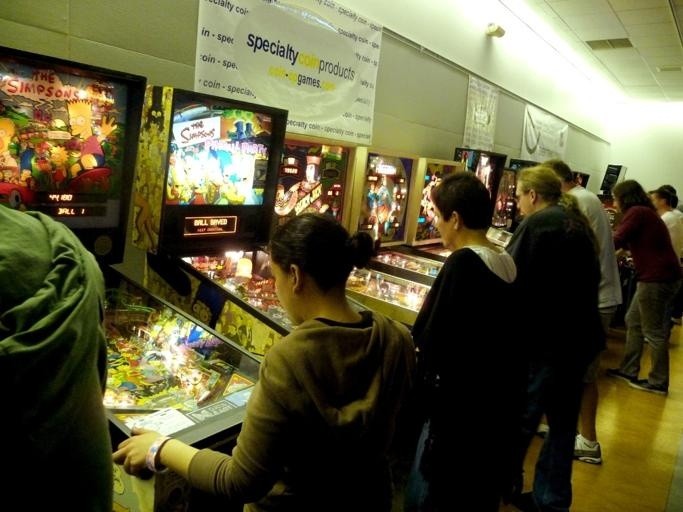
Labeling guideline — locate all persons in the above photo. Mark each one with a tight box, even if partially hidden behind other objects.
[507,160,623,510]
[609,179,683,395]
[112,211,416,512]
[410,170,522,511]
[1,203,113,512]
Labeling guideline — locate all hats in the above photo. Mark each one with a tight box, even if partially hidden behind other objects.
[650,184,676,197]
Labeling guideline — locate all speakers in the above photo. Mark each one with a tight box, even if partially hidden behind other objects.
[600,164,628,192]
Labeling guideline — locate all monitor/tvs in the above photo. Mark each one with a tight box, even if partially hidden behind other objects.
[0,58,130,231]
[474,153,500,198]
[357,152,412,243]
[417,163,456,243]
[164,97,276,208]
[272,139,349,239]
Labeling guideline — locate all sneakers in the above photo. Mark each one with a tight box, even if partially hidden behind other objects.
[573,436,602,464]
[514,491,572,512]
[628,379,668,396]
[536,424,550,438]
[606,368,639,382]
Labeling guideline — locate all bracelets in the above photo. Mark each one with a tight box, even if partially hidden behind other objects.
[145,436,171,475]
